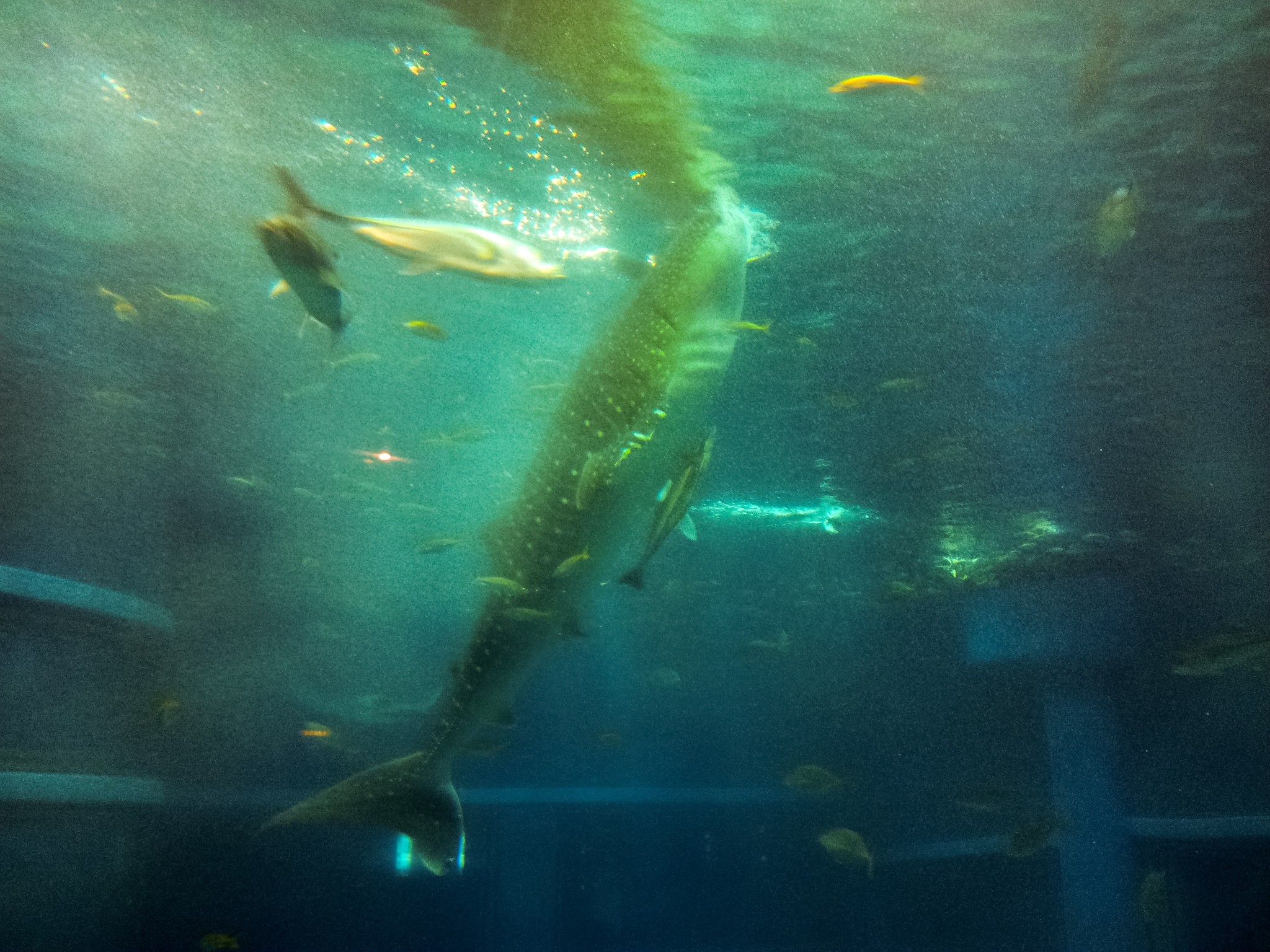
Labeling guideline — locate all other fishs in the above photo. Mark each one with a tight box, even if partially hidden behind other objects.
[88,63,1264,937]
